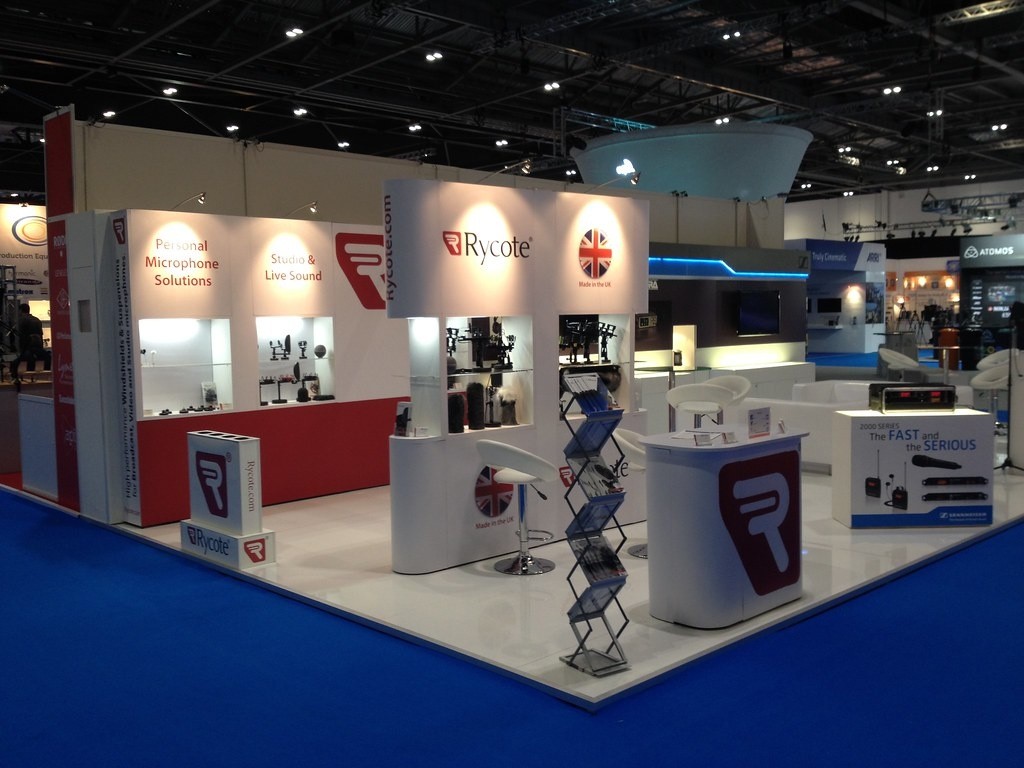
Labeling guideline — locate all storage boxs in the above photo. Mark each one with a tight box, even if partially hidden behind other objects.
[414,427,428,437]
[393,402,413,437]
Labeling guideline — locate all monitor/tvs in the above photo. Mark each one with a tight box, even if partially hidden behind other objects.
[736,289,782,337]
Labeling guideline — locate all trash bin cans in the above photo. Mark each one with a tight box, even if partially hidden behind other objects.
[960,328,983,372]
[937,327,960,370]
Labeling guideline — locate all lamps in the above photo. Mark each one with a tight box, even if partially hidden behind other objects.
[295,200,318,218]
[585,171,641,196]
[477,158,532,185]
[172,192,205,212]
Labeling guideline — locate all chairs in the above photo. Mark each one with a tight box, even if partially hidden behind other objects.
[612,429,649,559]
[666,375,752,429]
[970,349,1019,429]
[879,347,919,382]
[476,440,560,576]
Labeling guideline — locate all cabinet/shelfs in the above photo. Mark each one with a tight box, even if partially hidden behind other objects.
[136,317,334,417]
[388,314,649,575]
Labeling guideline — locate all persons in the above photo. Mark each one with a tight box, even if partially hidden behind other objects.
[18,304,43,361]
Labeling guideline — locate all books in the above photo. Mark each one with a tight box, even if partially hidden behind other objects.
[561,373,629,615]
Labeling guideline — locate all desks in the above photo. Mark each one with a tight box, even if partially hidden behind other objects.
[874,331,916,355]
